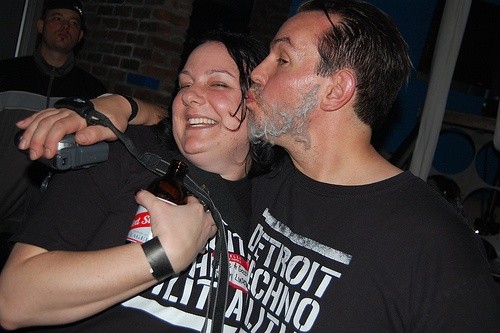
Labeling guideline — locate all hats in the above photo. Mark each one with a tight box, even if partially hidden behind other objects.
[48,1,85,27]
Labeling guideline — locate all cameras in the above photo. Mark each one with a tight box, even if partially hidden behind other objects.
[15,129,109,170]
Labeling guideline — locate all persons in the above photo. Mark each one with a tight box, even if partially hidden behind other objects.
[0,29,286,333]
[0,0,111,271]
[14,0,500,333]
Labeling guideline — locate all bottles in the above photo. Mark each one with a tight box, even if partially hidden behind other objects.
[124,159,189,245]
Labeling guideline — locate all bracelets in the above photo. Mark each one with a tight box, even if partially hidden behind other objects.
[121,93,139,122]
[141,237,175,282]
